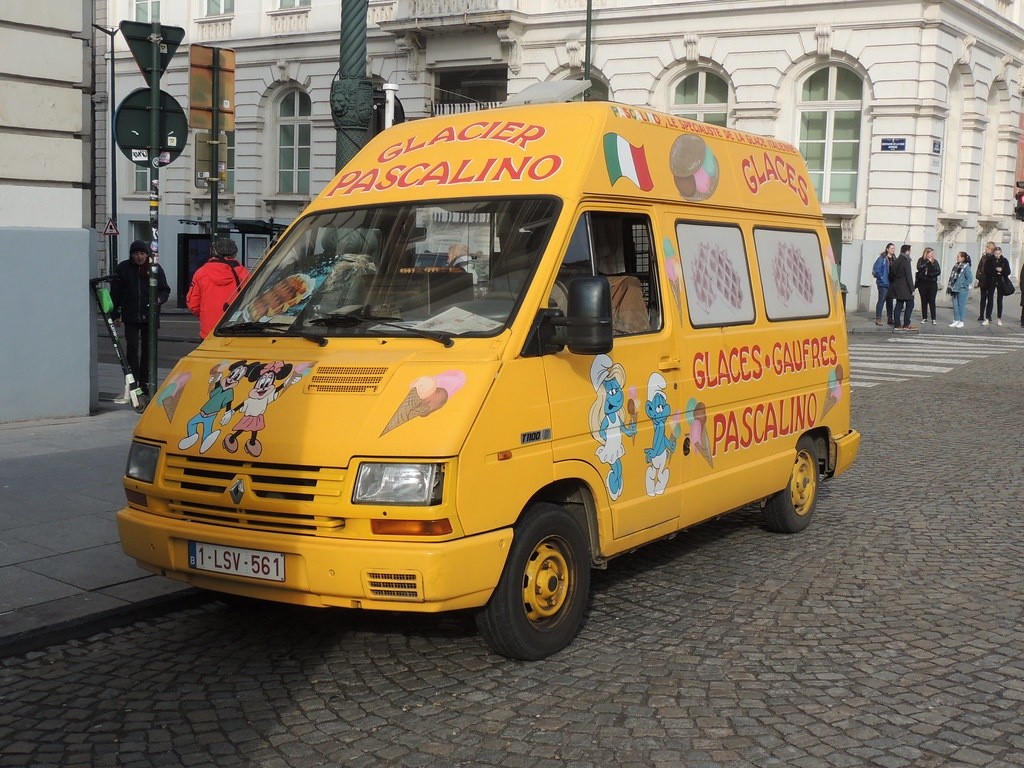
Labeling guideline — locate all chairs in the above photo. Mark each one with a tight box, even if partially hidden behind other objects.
[605,275,651,335]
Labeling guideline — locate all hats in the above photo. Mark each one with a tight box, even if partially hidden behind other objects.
[129,240,147,254]
[210,238,238,258]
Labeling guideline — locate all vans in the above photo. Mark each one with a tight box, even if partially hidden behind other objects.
[114,98,860,660]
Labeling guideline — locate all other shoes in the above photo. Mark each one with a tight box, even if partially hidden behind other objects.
[1021,322,1024,326]
[978,316,984,320]
[932,320,938,324]
[948,320,959,327]
[981,319,989,325]
[955,322,964,328]
[887,317,895,325]
[997,318,1002,326]
[875,318,883,326]
[139,386,149,396]
[989,317,992,321]
[894,325,903,331]
[903,324,918,330]
[921,319,928,323]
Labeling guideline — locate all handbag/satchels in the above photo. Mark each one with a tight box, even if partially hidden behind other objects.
[914,271,919,288]
[1002,276,1015,296]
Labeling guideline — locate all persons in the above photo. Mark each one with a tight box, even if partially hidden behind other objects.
[888,245,915,328]
[915,247,941,325]
[525,223,650,336]
[871,242,897,326]
[1020,263,1024,326]
[946,252,973,328]
[446,243,479,284]
[185,237,252,343]
[109,240,171,395]
[976,242,1011,326]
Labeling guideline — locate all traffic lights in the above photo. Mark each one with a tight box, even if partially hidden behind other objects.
[1014,181,1024,223]
